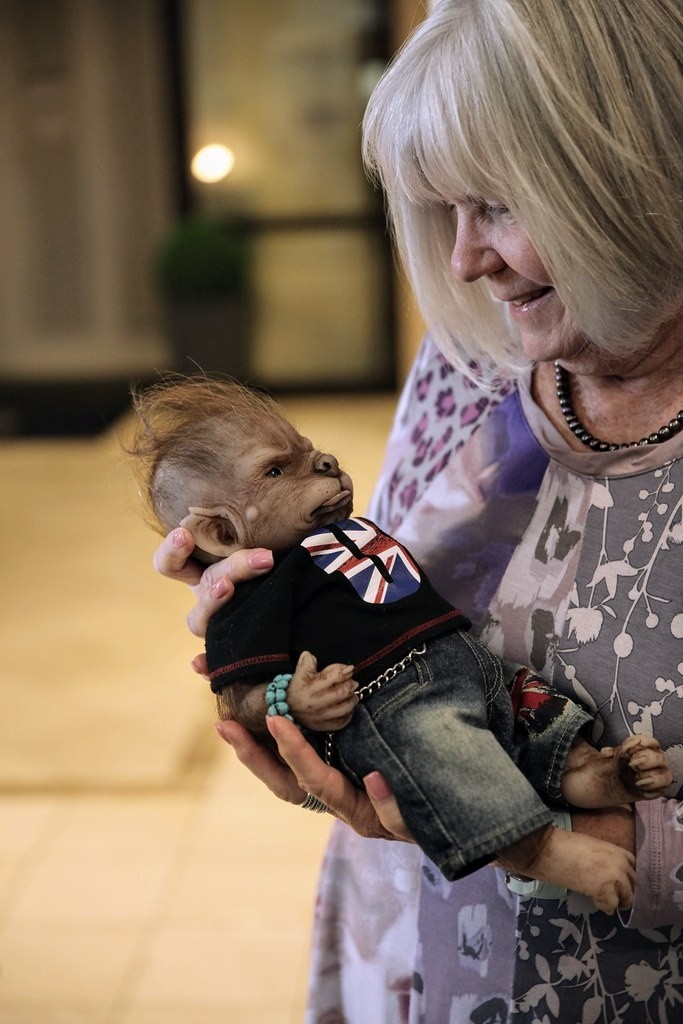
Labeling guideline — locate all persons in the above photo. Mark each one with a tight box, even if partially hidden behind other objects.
[152,1,683,1024]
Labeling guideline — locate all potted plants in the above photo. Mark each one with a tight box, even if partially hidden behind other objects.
[151,212,252,381]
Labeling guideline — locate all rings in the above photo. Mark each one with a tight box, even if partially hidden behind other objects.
[300,793,327,814]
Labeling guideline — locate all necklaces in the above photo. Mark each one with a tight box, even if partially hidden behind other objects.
[554,363,683,453]
